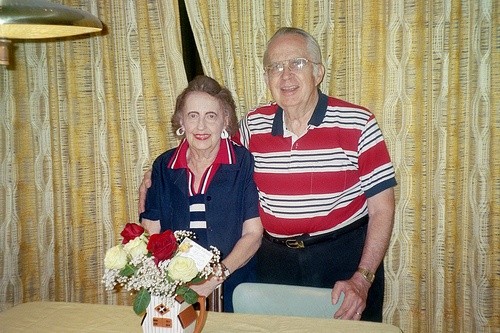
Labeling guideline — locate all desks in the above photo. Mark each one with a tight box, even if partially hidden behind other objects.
[0,301,402,333]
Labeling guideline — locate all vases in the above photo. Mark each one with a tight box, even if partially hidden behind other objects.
[141,294,206,333]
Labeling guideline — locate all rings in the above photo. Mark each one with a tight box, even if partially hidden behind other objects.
[355,312,361,316]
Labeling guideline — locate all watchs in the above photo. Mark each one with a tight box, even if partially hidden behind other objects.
[220,263,230,278]
[357,268,375,283]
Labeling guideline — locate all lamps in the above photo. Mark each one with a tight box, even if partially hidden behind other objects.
[0,0,103,70]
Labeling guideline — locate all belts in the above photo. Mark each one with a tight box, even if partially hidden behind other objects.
[263,213,369,249]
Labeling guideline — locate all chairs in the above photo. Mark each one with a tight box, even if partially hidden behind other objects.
[232,282,346,319]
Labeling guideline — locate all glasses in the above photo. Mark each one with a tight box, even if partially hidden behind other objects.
[263,58,318,74]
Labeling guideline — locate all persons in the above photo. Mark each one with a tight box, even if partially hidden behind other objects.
[138,27,398,323]
[138,75,264,313]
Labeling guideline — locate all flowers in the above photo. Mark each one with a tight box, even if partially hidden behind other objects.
[100,223,231,316]
